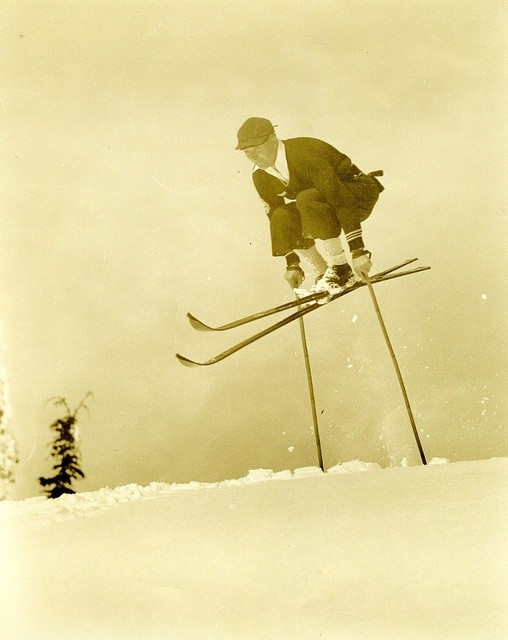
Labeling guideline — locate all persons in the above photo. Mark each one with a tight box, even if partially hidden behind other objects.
[235,116,384,291]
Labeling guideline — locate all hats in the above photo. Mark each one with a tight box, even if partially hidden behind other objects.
[235,117,274,151]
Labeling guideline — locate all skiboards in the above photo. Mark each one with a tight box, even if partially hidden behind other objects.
[177,259,433,366]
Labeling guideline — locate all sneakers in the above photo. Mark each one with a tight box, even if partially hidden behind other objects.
[318,263,353,292]
